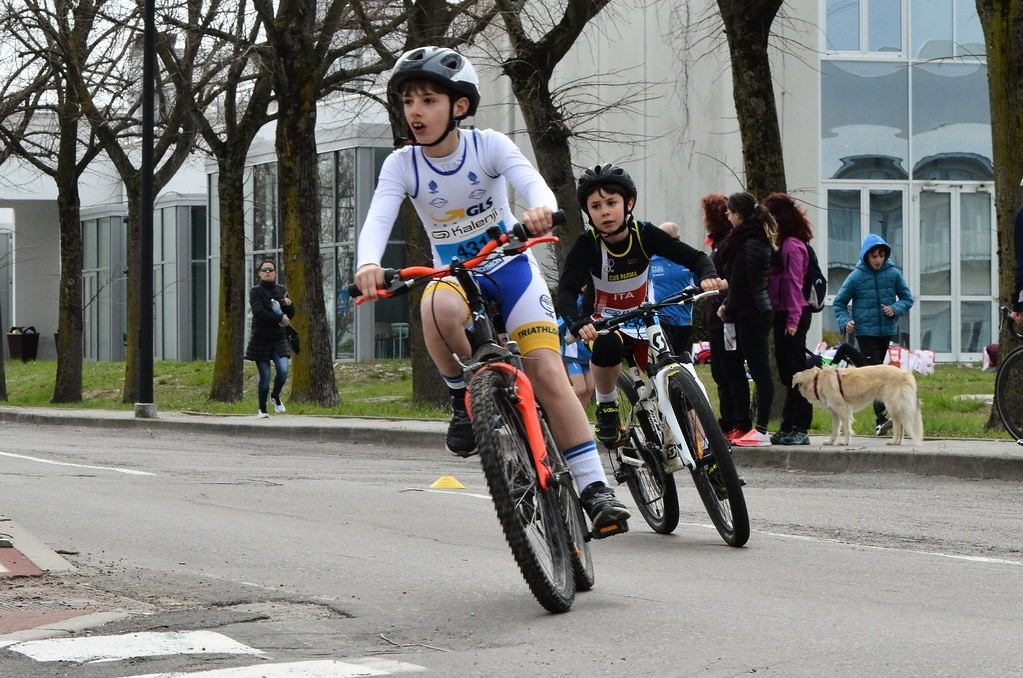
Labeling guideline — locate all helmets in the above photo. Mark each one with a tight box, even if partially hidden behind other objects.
[387,46,481,120]
[577,164,637,216]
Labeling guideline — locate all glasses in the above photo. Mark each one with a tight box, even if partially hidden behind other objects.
[725,210,735,216]
[260,267,275,272]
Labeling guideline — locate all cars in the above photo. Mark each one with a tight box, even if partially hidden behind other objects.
[391,323,410,339]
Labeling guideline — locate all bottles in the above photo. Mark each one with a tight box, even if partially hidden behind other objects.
[271,299,287,328]
[723,318,736,351]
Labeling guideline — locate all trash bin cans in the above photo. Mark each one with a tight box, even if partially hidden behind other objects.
[6,326,40,363]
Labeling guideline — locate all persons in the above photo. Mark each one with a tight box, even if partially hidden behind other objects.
[244,261,295,418]
[701,194,752,444]
[354,46,631,528]
[762,193,814,445]
[556,285,594,410]
[717,192,778,447]
[1010,178,1023,324]
[558,164,747,500]
[647,222,707,357]
[833,232,913,436]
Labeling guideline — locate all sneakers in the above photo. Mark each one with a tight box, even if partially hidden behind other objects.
[779,429,811,445]
[706,462,747,494]
[594,401,621,444]
[443,396,480,457]
[580,481,630,527]
[731,429,772,446]
[770,429,793,444]
[722,426,747,442]
[875,413,892,436]
[270,393,285,414]
[257,409,269,419]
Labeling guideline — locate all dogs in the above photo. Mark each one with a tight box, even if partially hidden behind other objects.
[792,364,925,446]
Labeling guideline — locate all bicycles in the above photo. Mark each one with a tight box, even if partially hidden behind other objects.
[347,208,629,615]
[564,278,750,547]
[995,306,1023,445]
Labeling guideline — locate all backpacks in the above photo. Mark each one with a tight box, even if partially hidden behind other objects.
[803,242,826,312]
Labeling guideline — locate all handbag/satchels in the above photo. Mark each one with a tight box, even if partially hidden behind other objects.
[983,345,997,372]
[690,340,711,362]
[812,340,827,356]
[285,325,300,355]
[882,345,936,377]
